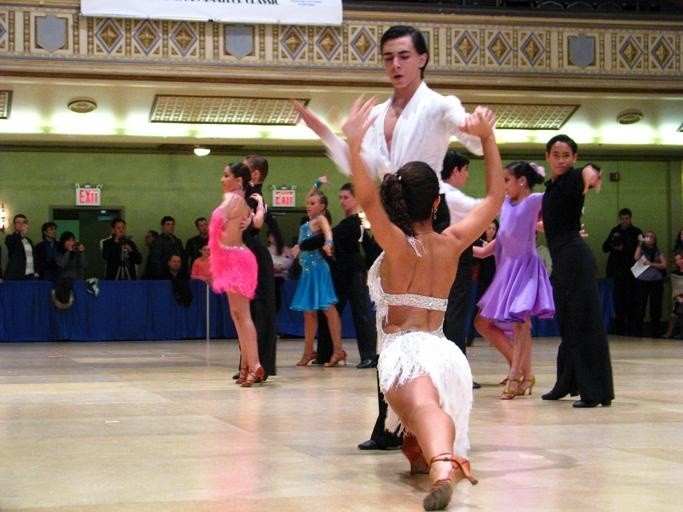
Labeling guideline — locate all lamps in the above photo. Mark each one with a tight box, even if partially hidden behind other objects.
[194,148,211,156]
[616,109,643,125]
[67,96,98,114]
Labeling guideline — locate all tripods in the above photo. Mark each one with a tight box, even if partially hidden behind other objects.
[115,267,131,280]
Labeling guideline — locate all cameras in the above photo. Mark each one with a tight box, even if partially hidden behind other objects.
[641,234,650,241]
[120,235,131,243]
[72,242,81,249]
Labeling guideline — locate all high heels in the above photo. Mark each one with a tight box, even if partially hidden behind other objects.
[518,376,535,396]
[401,433,477,511]
[500,375,523,399]
[297,349,347,368]
[232,359,270,386]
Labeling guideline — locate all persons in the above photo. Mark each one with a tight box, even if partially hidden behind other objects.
[287,24,497,452]
[264,227,305,277]
[3,214,85,281]
[477,220,498,294]
[232,154,277,380]
[437,149,489,388]
[289,174,348,368]
[340,93,507,512]
[289,181,380,368]
[602,208,682,339]
[99,217,142,280]
[140,215,211,306]
[471,160,555,401]
[535,134,616,408]
[189,160,266,388]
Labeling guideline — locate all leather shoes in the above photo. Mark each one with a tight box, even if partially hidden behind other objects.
[542,383,579,400]
[358,439,402,450]
[573,398,611,408]
[357,360,377,368]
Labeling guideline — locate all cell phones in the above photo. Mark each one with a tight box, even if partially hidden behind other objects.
[21,219,28,224]
